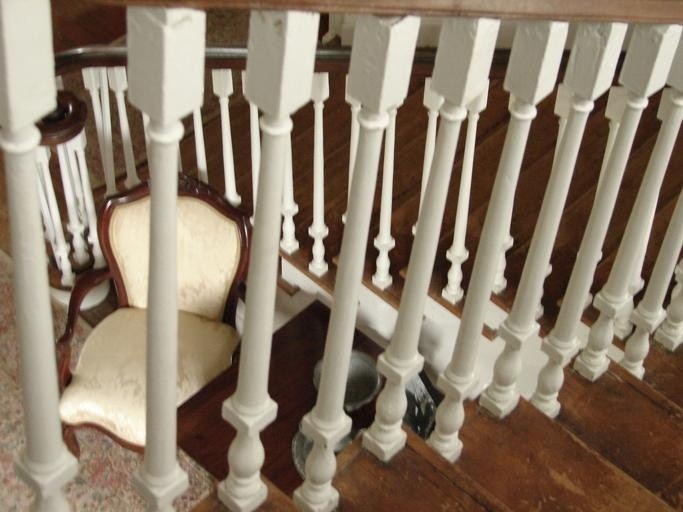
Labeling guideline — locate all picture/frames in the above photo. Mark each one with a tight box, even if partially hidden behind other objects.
[403,369,441,443]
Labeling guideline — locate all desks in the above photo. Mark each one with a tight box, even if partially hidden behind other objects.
[175,301,443,502]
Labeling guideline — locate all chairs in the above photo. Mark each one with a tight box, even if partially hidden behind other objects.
[54,169,252,463]
